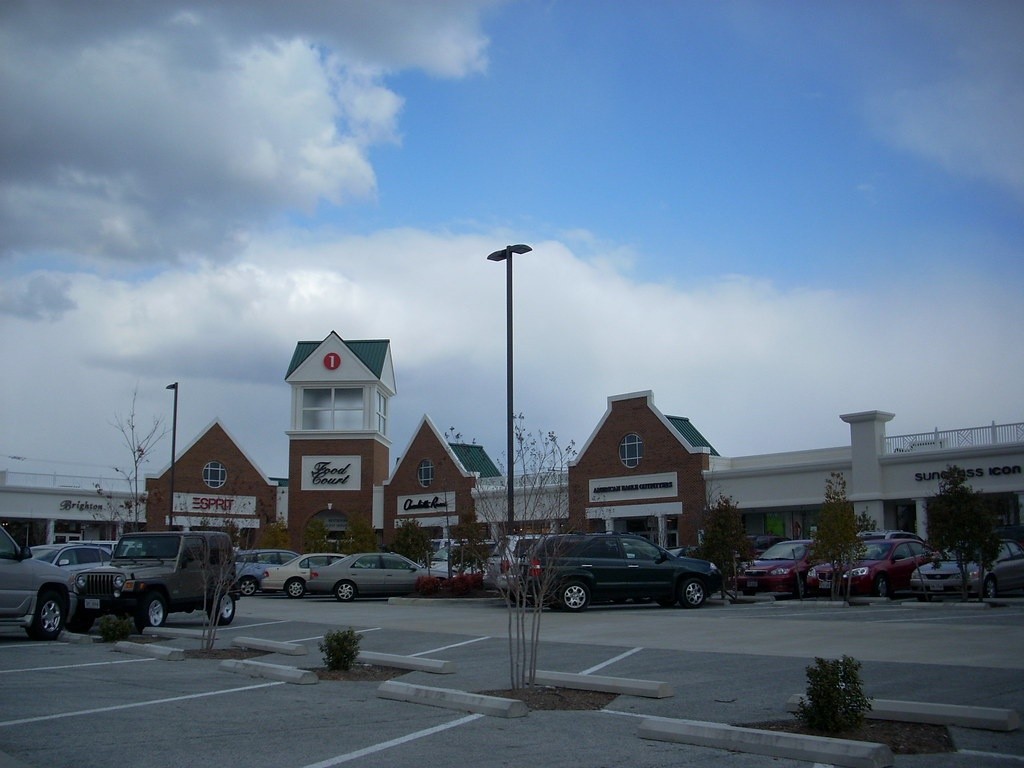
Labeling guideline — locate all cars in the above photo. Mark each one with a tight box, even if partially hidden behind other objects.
[306,552,458,603]
[68,540,143,556]
[422,538,497,577]
[807,538,940,599]
[234,549,311,596]
[0,525,79,640]
[737,540,831,600]
[726,535,792,590]
[909,539,1024,603]
[991,524,1024,547]
[855,530,925,542]
[260,552,364,599]
[483,534,604,608]
[30,544,113,572]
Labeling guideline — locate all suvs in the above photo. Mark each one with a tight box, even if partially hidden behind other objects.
[64,531,241,634]
[516,530,722,613]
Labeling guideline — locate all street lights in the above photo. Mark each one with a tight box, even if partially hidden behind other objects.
[487,244,533,535]
[166,382,178,531]
